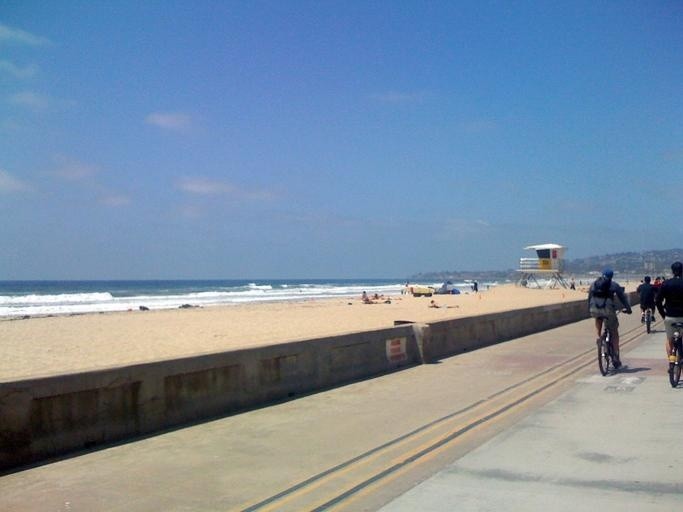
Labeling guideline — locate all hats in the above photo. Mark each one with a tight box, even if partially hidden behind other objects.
[602,268,613,278]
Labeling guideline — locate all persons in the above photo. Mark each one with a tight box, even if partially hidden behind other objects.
[637,276,669,322]
[570,278,575,289]
[654,262,683,372]
[405,281,409,287]
[362,291,385,304]
[472,280,478,292]
[587,267,632,368]
[429,300,460,308]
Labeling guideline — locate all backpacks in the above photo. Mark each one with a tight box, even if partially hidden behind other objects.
[594,276,611,299]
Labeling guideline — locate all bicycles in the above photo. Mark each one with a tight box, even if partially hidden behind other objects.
[664,322,682,389]
[594,307,625,378]
[643,308,653,335]
